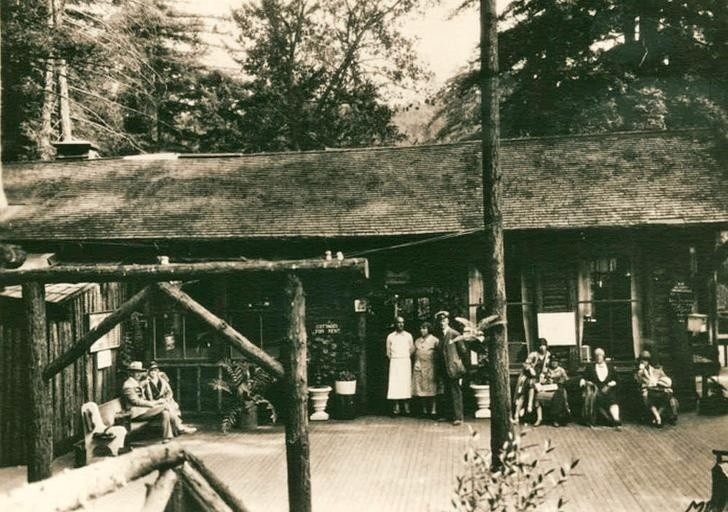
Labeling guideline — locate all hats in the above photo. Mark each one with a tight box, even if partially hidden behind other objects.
[437,313,449,321]
[147,361,163,370]
[127,361,148,372]
[549,353,560,362]
[637,351,651,360]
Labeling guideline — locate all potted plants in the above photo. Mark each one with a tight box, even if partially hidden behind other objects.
[307,332,337,422]
[207,355,279,432]
[465,326,493,418]
[334,369,357,397]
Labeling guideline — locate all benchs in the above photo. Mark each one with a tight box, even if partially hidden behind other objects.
[85,396,162,454]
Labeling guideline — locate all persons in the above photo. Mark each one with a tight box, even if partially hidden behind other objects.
[145,360,180,421]
[412,322,439,416]
[633,351,673,427]
[580,348,623,428]
[513,338,555,421]
[435,311,466,425]
[122,360,197,443]
[533,354,571,427]
[385,316,415,415]
